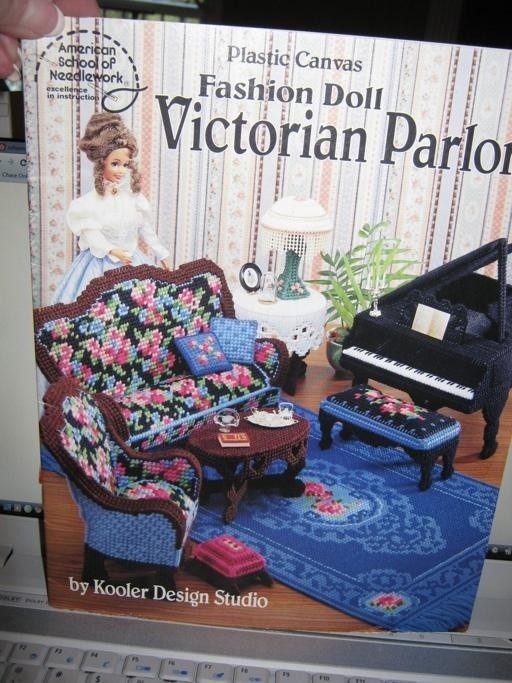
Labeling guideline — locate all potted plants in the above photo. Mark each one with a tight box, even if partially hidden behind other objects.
[305,219,418,381]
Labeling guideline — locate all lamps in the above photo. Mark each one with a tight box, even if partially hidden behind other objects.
[260,196,332,300]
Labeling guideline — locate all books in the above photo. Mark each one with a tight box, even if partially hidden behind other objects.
[216,432,249,448]
[20,16,512,631]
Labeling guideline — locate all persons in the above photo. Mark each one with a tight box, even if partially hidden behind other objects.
[0,0,107,80]
[53,110,173,305]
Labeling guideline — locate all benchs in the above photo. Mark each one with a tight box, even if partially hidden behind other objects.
[318,386,462,489]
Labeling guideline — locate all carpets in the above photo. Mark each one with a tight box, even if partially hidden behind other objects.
[190,399,499,633]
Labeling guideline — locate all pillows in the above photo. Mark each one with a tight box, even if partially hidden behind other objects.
[208,316,258,364]
[173,332,232,378]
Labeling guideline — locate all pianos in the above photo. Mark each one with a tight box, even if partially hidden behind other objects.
[339,237,511,459]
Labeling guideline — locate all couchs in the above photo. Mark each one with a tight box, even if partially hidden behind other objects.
[34,258,287,451]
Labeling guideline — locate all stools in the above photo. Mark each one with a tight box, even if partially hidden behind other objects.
[185,535,272,596]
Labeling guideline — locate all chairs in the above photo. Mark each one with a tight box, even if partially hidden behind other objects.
[184,410,309,520]
[38,379,204,599]
